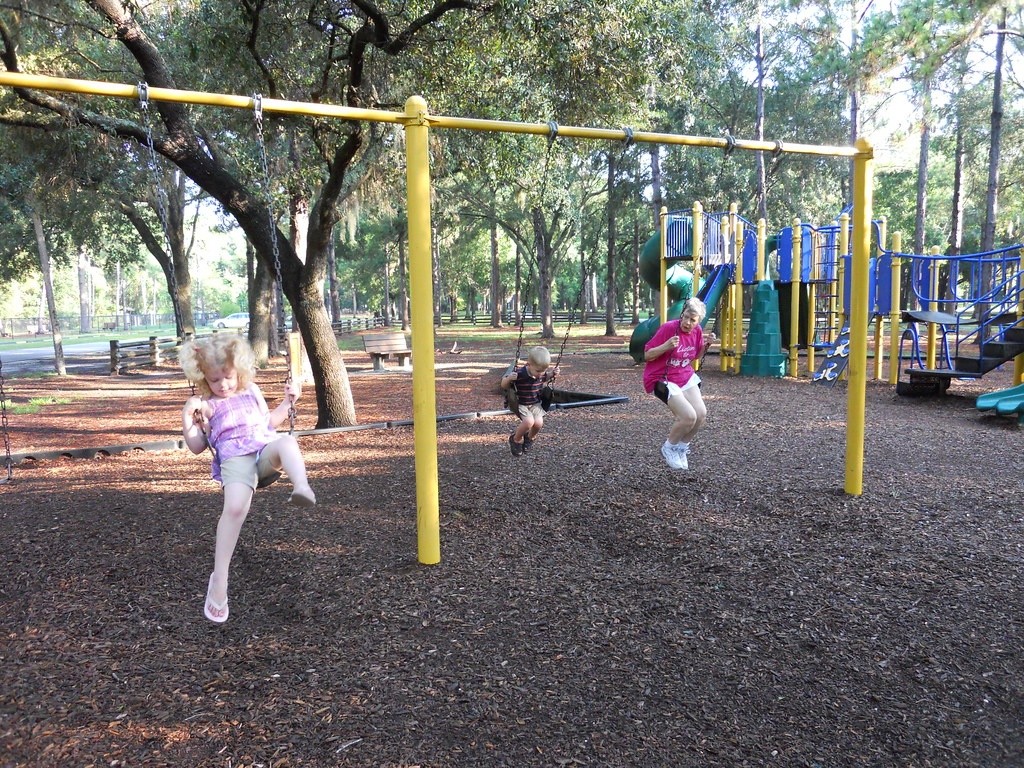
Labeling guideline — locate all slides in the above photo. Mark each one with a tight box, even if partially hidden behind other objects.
[811,311,859,387]
[630,214,709,365]
[691,262,728,353]
[977,380,1024,415]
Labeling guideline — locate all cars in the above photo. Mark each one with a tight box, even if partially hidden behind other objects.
[284,316,292,329]
[212,313,251,329]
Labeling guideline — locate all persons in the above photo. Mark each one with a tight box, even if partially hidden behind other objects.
[643,298,716,469]
[179,333,316,622]
[502,346,560,456]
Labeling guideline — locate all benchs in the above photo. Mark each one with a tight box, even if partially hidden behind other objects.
[361,333,413,371]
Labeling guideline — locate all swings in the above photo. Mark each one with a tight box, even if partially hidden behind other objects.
[655,153,783,400]
[502,144,629,422]
[139,100,295,490]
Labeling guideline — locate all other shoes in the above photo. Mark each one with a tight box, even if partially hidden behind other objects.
[523,432,534,453]
[509,434,523,457]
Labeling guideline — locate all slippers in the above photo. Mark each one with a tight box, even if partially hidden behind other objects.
[204,572,229,623]
[287,487,316,508]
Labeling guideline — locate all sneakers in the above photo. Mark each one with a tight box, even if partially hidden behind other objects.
[661,444,682,469]
[678,446,691,470]
